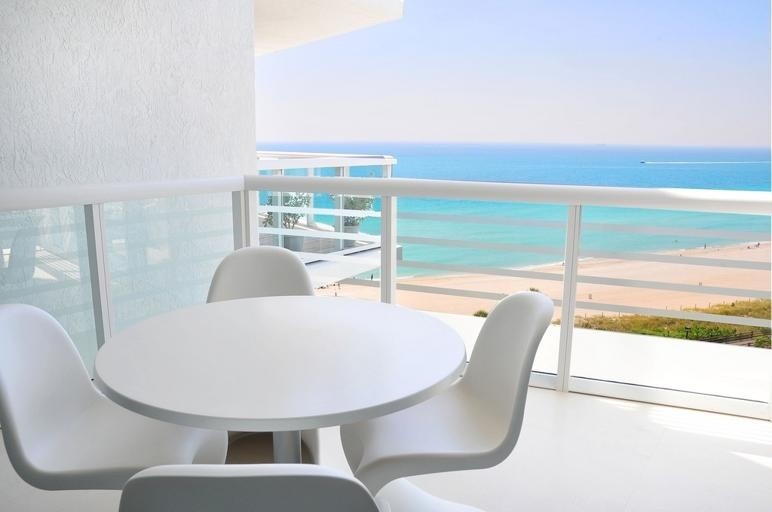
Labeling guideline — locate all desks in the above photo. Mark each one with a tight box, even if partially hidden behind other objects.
[91,294,467,463]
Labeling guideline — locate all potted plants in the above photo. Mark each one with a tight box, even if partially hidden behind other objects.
[331,193,375,248]
[261,192,313,250]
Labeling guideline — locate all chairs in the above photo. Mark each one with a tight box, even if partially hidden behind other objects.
[340,290,556,498]
[118,463,382,512]
[1,303,203,491]
[206,245,314,302]
